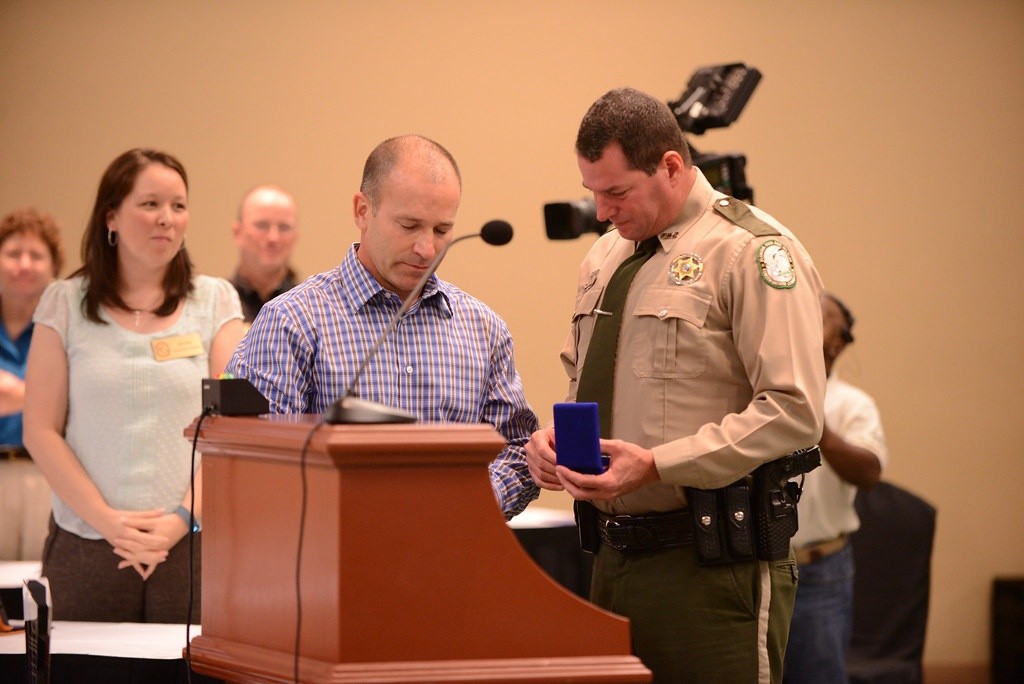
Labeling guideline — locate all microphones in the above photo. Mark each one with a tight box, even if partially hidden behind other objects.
[330,220,513,425]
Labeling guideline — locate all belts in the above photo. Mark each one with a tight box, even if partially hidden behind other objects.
[796,534,850,567]
[0,450,31,461]
[593,511,699,560]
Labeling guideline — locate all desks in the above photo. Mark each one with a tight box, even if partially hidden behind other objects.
[1,618,202,661]
[505,507,576,531]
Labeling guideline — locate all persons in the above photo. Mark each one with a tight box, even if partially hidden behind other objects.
[783,289,887,684]
[523,88,829,684]
[226,186,301,326]
[0,206,64,560]
[220,134,542,524]
[21,149,245,684]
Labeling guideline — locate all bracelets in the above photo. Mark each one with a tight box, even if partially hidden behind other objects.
[174,505,200,535]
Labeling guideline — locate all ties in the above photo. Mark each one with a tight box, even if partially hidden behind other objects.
[575,234,660,466]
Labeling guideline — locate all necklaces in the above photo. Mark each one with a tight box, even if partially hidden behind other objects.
[121,294,164,328]
